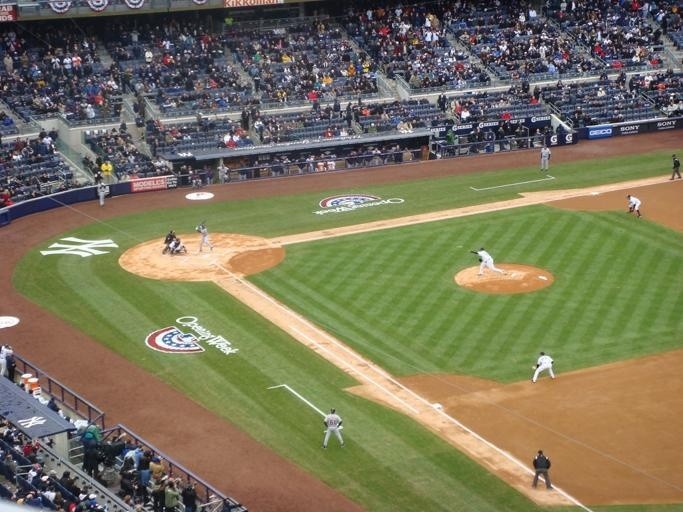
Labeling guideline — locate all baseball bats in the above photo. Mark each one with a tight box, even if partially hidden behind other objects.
[195,220,206,231]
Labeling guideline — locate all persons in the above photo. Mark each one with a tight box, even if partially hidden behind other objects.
[1,343,242,511]
[627,194,641,218]
[532,450,554,489]
[470,248,506,275]
[162,230,188,255]
[195,223,213,252]
[532,352,555,383]
[1,0,682,207]
[322,408,345,449]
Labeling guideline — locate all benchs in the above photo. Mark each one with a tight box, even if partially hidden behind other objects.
[0,437,78,511]
